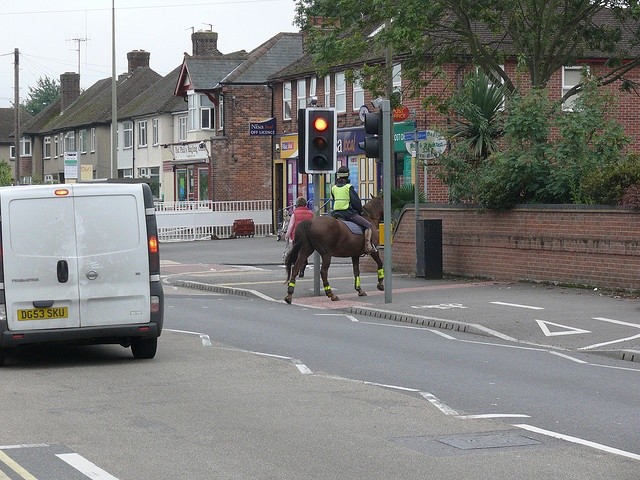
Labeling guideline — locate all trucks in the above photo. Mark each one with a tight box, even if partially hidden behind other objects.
[0,178,164,367]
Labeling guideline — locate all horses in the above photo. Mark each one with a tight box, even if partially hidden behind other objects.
[284,193,384,305]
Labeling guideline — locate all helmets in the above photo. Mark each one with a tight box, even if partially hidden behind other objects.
[337,166,350,178]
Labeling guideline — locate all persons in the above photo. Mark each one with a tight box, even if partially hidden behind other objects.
[331,166,374,253]
[286,197,313,276]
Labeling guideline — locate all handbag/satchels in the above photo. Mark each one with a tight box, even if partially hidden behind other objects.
[281,236,293,260]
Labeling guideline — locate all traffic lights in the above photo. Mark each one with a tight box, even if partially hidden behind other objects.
[298,109,305,174]
[305,107,337,174]
[358,113,382,163]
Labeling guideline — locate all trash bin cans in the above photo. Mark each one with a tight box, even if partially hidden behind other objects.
[379,222,396,245]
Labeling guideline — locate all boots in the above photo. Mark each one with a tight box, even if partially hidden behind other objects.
[299,269,305,278]
[364,228,375,252]
